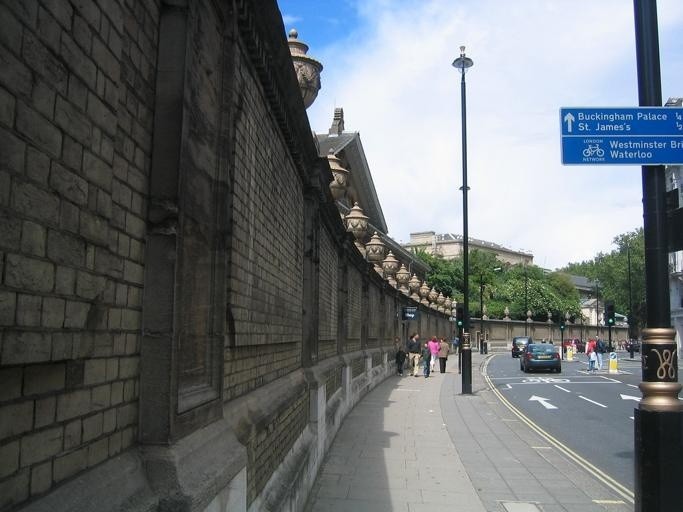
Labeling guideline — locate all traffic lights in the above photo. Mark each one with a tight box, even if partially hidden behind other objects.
[561,322,564,330]
[607,305,614,324]
[457,307,463,326]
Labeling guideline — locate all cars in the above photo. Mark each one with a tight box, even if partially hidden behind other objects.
[596,342,615,353]
[518,343,561,373]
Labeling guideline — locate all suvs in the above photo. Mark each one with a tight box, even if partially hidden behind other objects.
[625,339,642,352]
[564,337,584,352]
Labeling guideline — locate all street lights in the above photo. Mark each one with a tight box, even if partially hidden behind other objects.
[479,268,502,353]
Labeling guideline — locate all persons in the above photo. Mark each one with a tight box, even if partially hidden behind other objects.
[566,334,635,372]
[548,338,553,344]
[452,334,460,355]
[395,333,449,378]
[542,339,546,344]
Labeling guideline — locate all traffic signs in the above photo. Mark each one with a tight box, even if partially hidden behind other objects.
[551,103,683,165]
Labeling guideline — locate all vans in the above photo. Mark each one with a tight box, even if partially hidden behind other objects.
[512,336,533,357]
